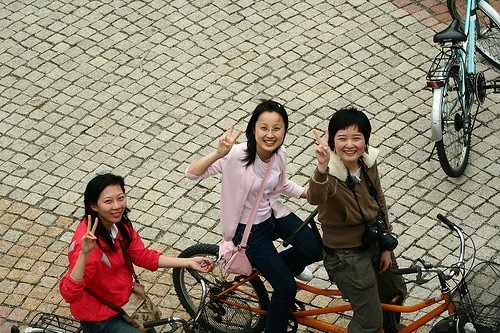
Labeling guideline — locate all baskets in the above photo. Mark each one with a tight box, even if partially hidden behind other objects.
[202,296,252,333]
[448,261,500,333]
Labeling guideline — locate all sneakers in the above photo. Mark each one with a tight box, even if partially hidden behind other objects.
[295,267,313,281]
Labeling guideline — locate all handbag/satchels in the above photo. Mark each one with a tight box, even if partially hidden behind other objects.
[218,240,253,276]
[120,281,162,333]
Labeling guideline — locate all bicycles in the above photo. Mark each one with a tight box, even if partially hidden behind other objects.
[23,267,215,333]
[426,1,500,177]
[173,212,500,333]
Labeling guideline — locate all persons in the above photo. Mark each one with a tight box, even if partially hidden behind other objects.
[184,101,323,333]
[58,172,215,333]
[307,107,408,333]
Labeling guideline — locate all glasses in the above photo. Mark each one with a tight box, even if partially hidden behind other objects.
[254,124,285,132]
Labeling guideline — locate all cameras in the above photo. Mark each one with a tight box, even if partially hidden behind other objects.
[361,221,398,251]
